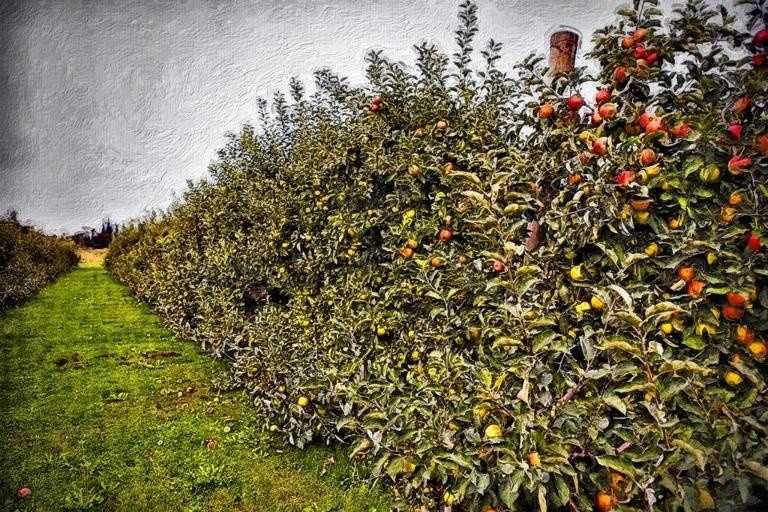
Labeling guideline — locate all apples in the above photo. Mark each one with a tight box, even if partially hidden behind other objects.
[279,27,768,512]
[17,487,32,498]
